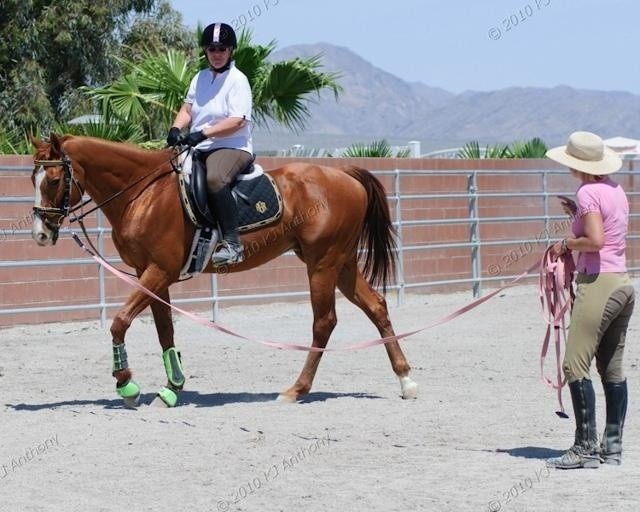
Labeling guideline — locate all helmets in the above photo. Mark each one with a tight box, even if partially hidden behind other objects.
[201,24,237,48]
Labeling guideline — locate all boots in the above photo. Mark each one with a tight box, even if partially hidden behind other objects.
[209,185,246,263]
[597,378,628,464]
[546,377,601,468]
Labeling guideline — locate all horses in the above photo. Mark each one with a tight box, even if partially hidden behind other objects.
[29,129,418,407]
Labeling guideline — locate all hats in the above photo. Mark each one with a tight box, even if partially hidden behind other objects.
[545,131,622,176]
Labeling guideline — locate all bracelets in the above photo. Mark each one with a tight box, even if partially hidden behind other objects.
[562,238,571,253]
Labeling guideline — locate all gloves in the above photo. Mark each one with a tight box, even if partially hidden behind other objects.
[167,127,182,145]
[182,131,207,146]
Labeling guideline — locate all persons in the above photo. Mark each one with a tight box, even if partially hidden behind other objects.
[166,22,257,267]
[545,132,637,471]
